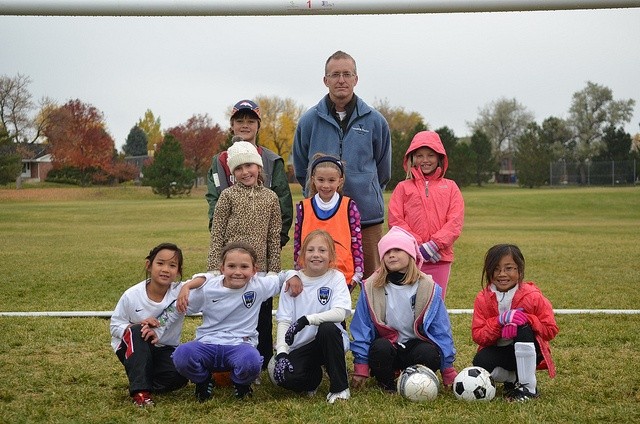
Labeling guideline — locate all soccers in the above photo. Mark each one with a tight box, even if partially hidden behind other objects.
[397,364,440,402]
[453,366,496,402]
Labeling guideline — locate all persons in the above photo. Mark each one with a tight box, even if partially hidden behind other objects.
[109,244,216,408]
[294,51,391,331]
[387,129,465,302]
[139,246,302,401]
[292,154,367,325]
[269,230,353,403]
[203,100,292,254]
[206,137,281,369]
[354,225,462,391]
[470,244,560,404]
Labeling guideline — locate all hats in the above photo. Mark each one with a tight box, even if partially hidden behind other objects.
[227,136,262,174]
[377,226,424,270]
[230,100,261,120]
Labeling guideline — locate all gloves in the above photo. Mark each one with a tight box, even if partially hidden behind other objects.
[430,253,440,264]
[285,316,307,345]
[500,308,527,325]
[274,356,294,383]
[502,323,517,338]
[420,240,438,262]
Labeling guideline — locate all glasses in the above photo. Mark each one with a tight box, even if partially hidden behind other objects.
[493,265,518,272]
[327,72,356,78]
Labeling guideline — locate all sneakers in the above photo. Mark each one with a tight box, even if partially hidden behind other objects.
[195,379,215,402]
[503,371,518,398]
[232,381,252,400]
[132,391,156,408]
[328,389,351,405]
[308,366,323,397]
[506,384,538,402]
